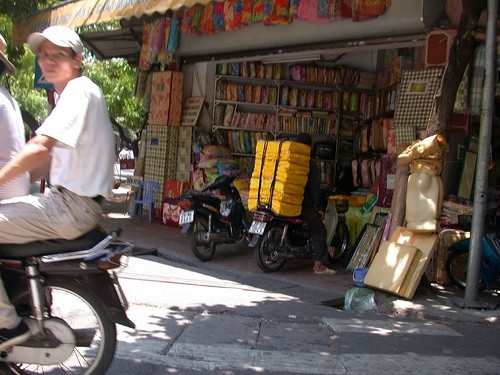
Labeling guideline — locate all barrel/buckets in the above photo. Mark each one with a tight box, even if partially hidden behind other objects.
[352,267,368,287]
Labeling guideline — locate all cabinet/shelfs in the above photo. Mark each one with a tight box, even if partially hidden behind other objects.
[211,60,405,212]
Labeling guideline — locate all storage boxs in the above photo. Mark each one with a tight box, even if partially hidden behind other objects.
[141,126,180,213]
[160,179,191,228]
[148,69,184,129]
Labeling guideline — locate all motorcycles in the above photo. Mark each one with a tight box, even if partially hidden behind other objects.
[177,170,251,261]
[0,224,139,374]
[445,191,500,291]
[249,186,349,273]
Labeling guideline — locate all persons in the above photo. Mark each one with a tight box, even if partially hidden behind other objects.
[294,132,338,275]
[0,34,32,202]
[0,24,117,345]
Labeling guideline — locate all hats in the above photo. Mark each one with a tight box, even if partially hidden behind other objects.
[28,25,84,58]
[0,34,16,75]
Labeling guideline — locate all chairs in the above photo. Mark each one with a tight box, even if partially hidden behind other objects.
[129,181,162,225]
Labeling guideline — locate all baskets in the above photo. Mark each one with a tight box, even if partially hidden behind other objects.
[336,199,349,213]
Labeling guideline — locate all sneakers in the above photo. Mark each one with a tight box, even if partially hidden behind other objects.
[0,321,32,350]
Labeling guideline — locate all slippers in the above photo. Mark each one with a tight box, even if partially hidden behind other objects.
[266,258,276,264]
[314,267,337,275]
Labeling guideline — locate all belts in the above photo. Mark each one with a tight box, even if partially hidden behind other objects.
[93,195,105,204]
